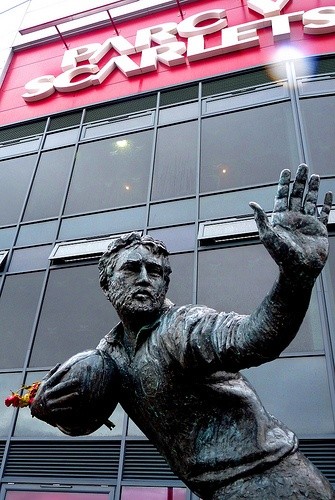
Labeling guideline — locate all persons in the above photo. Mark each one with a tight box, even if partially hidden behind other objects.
[33,163,334,499]
